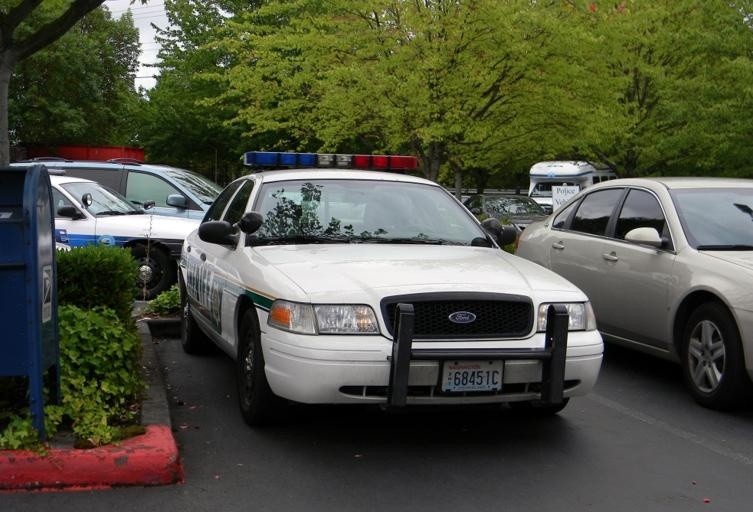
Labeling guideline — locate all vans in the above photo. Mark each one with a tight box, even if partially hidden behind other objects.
[527,162,614,213]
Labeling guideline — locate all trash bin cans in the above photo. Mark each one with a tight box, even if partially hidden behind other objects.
[1,164,62,441]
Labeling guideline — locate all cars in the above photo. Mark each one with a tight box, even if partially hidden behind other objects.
[12,162,228,219]
[512,175,751,414]
[43,175,211,298]
[464,192,554,244]
[178,152,603,434]
